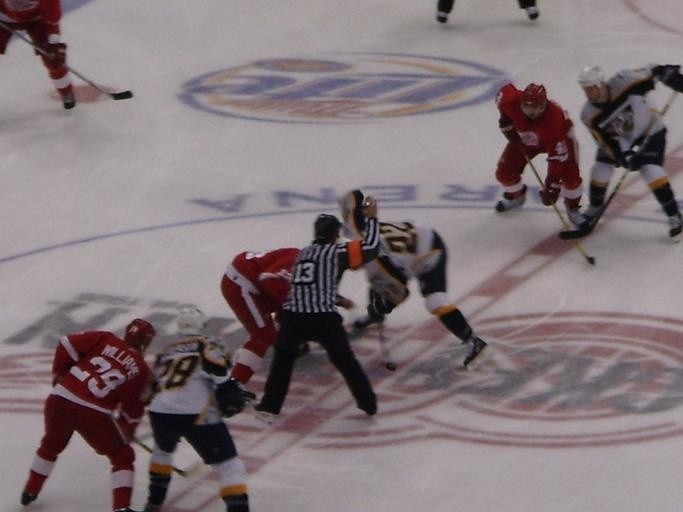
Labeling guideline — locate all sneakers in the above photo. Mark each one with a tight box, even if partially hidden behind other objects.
[567,205,586,227]
[437,11,447,22]
[668,211,682,238]
[584,205,603,218]
[525,7,538,19]
[353,310,379,328]
[464,335,487,366]
[63,93,76,108]
[495,196,526,212]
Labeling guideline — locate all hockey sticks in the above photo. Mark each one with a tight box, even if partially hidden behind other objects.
[0,22,134,100]
[522,153,596,267]
[557,90,679,240]
[128,439,199,477]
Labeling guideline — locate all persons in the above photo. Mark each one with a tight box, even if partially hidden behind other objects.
[143,307,251,512]
[0,0,77,111]
[436,0,539,23]
[494,83,586,226]
[219,247,354,394]
[253,195,380,422]
[18,318,157,512]
[576,63,683,245]
[339,188,492,370]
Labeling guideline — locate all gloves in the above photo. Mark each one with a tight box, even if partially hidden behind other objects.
[496,119,520,145]
[539,179,561,206]
[657,63,683,93]
[618,150,641,171]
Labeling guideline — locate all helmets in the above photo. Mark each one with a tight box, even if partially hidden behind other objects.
[520,83,546,107]
[577,65,606,86]
[176,307,207,337]
[124,318,155,351]
